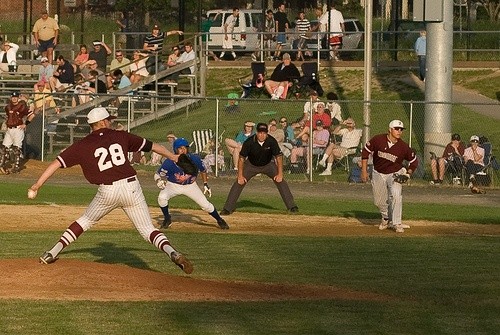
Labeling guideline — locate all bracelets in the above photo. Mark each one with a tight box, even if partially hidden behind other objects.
[25,119,31,126]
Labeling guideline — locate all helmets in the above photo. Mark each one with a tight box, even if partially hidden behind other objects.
[173,138,189,154]
[11,92,19,98]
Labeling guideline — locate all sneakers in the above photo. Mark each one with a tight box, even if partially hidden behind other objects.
[288,206,299,214]
[319,169,331,176]
[470,174,476,183]
[36,55,42,60]
[171,251,193,274]
[393,224,404,232]
[318,160,325,168]
[469,182,473,188]
[218,218,229,230]
[39,250,59,264]
[429,178,444,186]
[379,218,390,231]
[161,218,172,231]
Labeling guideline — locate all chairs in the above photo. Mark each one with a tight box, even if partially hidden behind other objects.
[463,141,498,188]
[290,62,318,98]
[330,140,363,173]
[237,61,267,98]
[189,129,225,172]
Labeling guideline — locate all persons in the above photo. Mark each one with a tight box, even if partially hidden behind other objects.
[218,7,240,60]
[268,87,364,177]
[461,134,487,190]
[198,13,220,62]
[26,107,195,275]
[60,8,196,117]
[262,52,301,101]
[151,137,229,231]
[218,121,301,217]
[428,132,467,188]
[150,130,179,164]
[32,9,59,64]
[0,38,20,76]
[360,118,419,234]
[250,0,347,62]
[224,118,257,173]
[16,55,59,122]
[0,90,37,177]
[415,27,428,84]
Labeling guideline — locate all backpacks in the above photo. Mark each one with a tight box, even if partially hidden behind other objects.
[349,164,362,183]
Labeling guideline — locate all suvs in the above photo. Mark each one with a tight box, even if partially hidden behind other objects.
[305,18,364,59]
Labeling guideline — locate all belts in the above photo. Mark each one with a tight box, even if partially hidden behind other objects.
[9,126,17,129]
[104,177,136,185]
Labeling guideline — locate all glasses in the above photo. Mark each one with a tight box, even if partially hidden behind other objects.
[117,55,122,57]
[393,128,403,131]
[281,120,287,123]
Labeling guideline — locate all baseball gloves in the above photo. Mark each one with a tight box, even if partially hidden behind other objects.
[394,173,410,184]
[176,154,197,175]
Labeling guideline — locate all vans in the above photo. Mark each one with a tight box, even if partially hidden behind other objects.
[208,10,265,60]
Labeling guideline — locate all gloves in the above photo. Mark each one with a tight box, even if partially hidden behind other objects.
[156,178,166,190]
[394,172,410,184]
[203,182,212,198]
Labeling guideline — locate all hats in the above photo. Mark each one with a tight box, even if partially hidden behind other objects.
[470,135,479,142]
[388,120,405,129]
[452,134,460,139]
[257,123,268,132]
[283,53,290,60]
[309,90,318,95]
[153,24,159,30]
[86,107,117,124]
[41,57,48,63]
[316,121,322,125]
[37,81,45,85]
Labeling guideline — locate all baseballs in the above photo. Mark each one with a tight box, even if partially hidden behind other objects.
[28,191,35,198]
